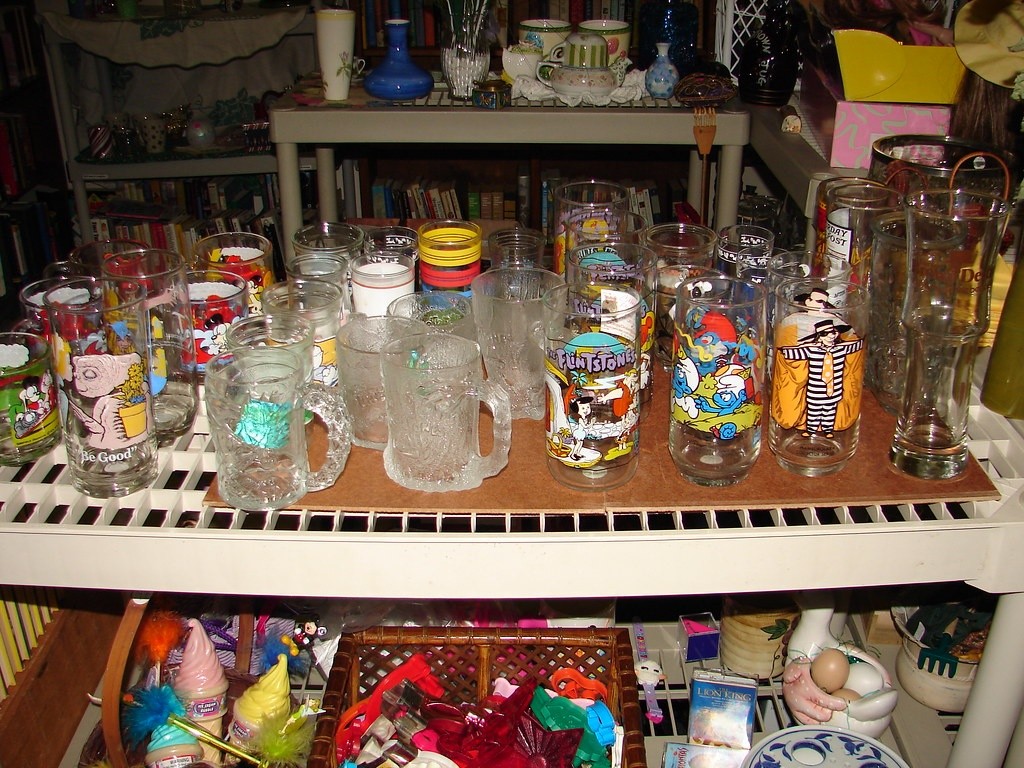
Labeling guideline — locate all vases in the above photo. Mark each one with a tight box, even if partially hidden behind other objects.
[434,0,496,101]
[638,0,699,78]
[363,18,433,100]
[739,0,800,107]
[645,42,680,101]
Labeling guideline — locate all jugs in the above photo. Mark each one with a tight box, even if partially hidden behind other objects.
[535,57,633,97]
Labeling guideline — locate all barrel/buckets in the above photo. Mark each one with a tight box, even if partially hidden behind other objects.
[866,134,1017,214]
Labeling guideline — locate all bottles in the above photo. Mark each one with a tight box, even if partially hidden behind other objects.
[645,42,679,99]
[363,20,434,100]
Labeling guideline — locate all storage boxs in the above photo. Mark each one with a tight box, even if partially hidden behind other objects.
[801,58,955,169]
[677,612,721,663]
[831,28,965,106]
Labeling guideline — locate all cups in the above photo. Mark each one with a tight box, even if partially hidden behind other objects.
[517,20,572,58]
[551,32,607,69]
[0,180,1014,513]
[316,9,356,101]
[577,18,631,67]
[87,112,166,162]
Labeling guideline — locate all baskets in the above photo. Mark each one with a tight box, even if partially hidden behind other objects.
[80,596,303,768]
[306,623,646,768]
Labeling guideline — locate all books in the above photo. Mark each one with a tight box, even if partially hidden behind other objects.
[0,0,686,284]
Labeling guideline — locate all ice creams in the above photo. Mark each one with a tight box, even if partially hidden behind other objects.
[146,619,289,768]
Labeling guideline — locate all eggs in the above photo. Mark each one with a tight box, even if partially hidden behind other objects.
[812,648,884,701]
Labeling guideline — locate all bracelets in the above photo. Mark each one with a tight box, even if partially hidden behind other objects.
[936,27,944,38]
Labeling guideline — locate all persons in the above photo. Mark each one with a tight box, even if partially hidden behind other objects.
[819,0,1024,194]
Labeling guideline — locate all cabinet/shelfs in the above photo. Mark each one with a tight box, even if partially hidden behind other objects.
[0,0,1023,768]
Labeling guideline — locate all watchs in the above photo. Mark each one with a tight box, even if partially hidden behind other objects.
[633,615,665,724]
